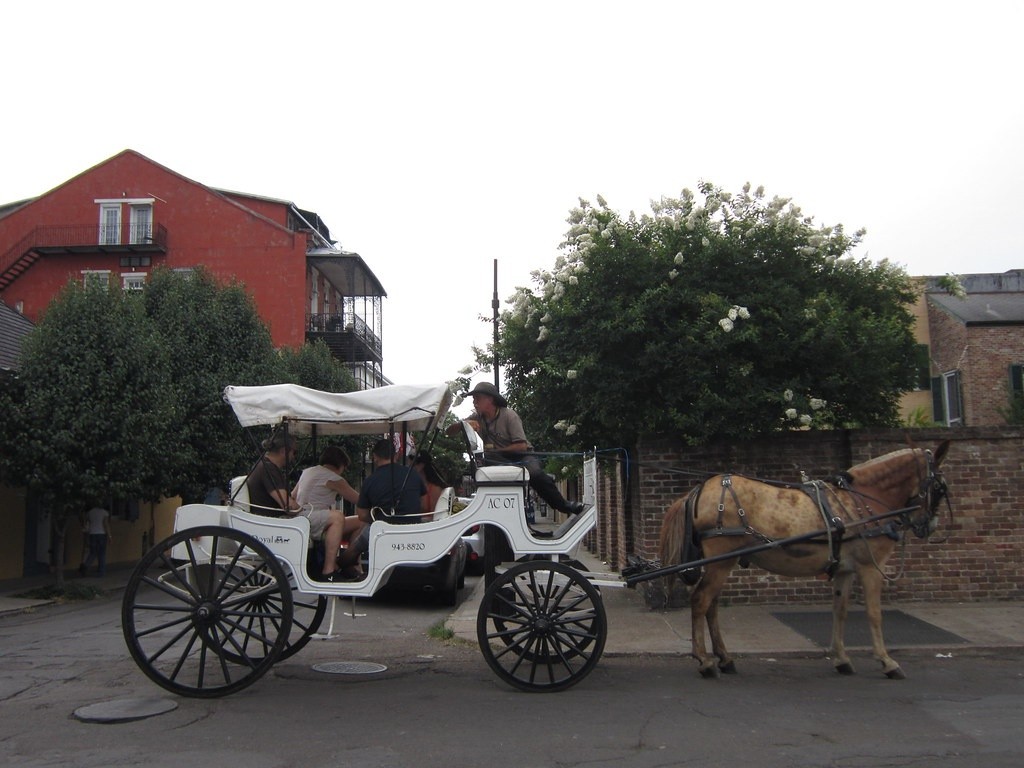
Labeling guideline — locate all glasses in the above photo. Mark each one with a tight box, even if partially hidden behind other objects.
[290,447,298,455]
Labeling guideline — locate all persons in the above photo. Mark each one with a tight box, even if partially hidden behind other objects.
[444,382,585,516]
[290,447,363,577]
[408,449,442,523]
[248,432,345,581]
[337,438,427,569]
[79,497,113,577]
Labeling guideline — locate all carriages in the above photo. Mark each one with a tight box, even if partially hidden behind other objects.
[120,377,957,695]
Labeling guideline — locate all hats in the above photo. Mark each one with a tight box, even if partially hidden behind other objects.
[460,382,508,407]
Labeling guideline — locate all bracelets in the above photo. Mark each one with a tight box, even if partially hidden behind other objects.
[109,536,112,538]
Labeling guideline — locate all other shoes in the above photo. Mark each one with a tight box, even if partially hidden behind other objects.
[570,501,585,514]
[319,571,360,582]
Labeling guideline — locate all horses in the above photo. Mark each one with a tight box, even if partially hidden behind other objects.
[658,431,952,680]
